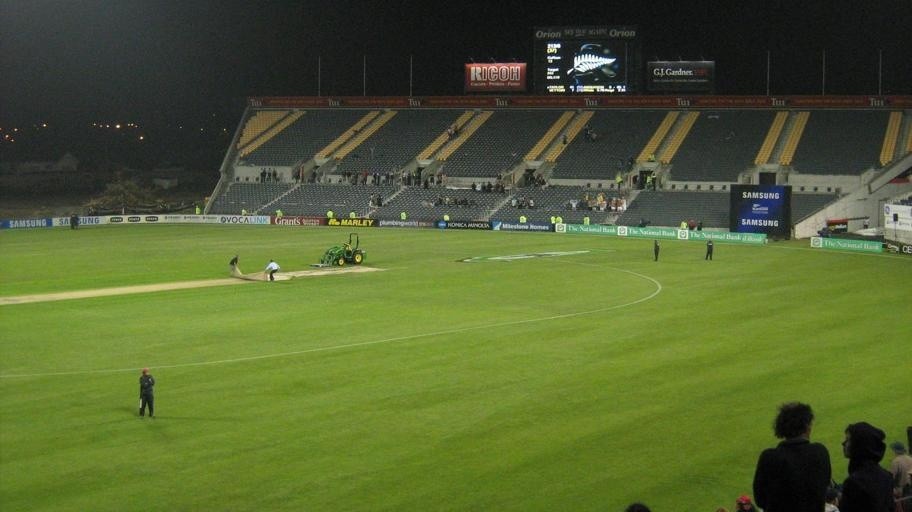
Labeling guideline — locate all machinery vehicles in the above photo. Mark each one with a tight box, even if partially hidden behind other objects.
[319,231,367,269]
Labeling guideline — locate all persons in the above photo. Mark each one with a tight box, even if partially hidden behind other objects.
[443,212,450,221]
[228,253,241,277]
[681,220,687,228]
[550,214,556,224]
[615,174,624,188]
[560,134,569,145]
[705,239,714,261]
[583,213,590,225]
[519,213,526,223]
[349,209,355,218]
[256,164,442,190]
[368,191,375,206]
[264,258,280,282]
[688,219,697,229]
[556,214,563,224]
[376,194,383,207]
[714,396,912,512]
[137,367,157,420]
[862,215,871,229]
[275,208,284,217]
[454,124,460,138]
[368,143,378,162]
[400,209,407,220]
[241,207,247,216]
[654,239,660,261]
[447,125,454,141]
[630,151,660,192]
[431,174,547,209]
[569,191,625,211]
[326,208,334,218]
[69,212,79,230]
[583,120,598,143]
[195,205,201,215]
